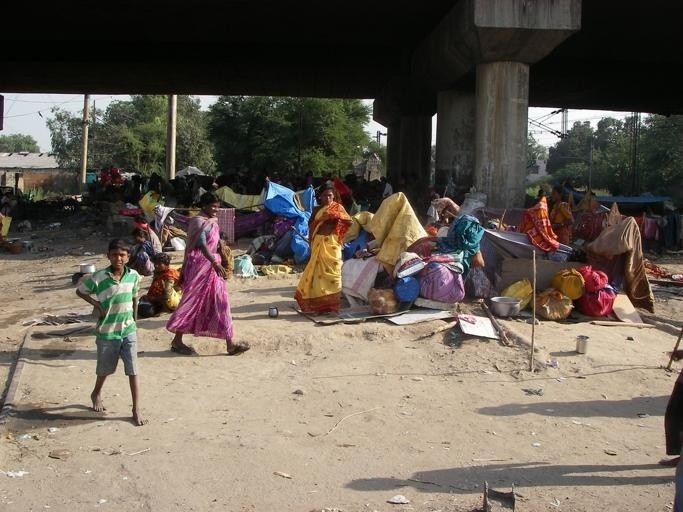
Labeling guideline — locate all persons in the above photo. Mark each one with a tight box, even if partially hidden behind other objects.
[138,179,174,251]
[191,167,393,207]
[134,216,163,255]
[75,238,149,426]
[549,185,575,245]
[140,252,180,317]
[165,190,250,357]
[0,191,12,217]
[173,229,234,292]
[292,182,355,318]
[125,227,155,277]
[657,349,682,466]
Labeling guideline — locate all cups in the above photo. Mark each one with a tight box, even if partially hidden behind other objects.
[575,335,590,355]
[270,306,278,317]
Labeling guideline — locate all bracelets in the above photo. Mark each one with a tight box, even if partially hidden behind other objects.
[211,260,219,269]
[557,224,560,228]
[560,222,565,226]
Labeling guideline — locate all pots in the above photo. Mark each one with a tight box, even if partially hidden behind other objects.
[490,297,524,317]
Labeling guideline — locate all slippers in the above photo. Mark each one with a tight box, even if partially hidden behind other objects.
[167,343,251,358]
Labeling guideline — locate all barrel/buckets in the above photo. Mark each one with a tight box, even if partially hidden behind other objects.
[395,276,419,301]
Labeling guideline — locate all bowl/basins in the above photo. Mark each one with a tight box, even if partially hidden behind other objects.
[20,241,32,248]
